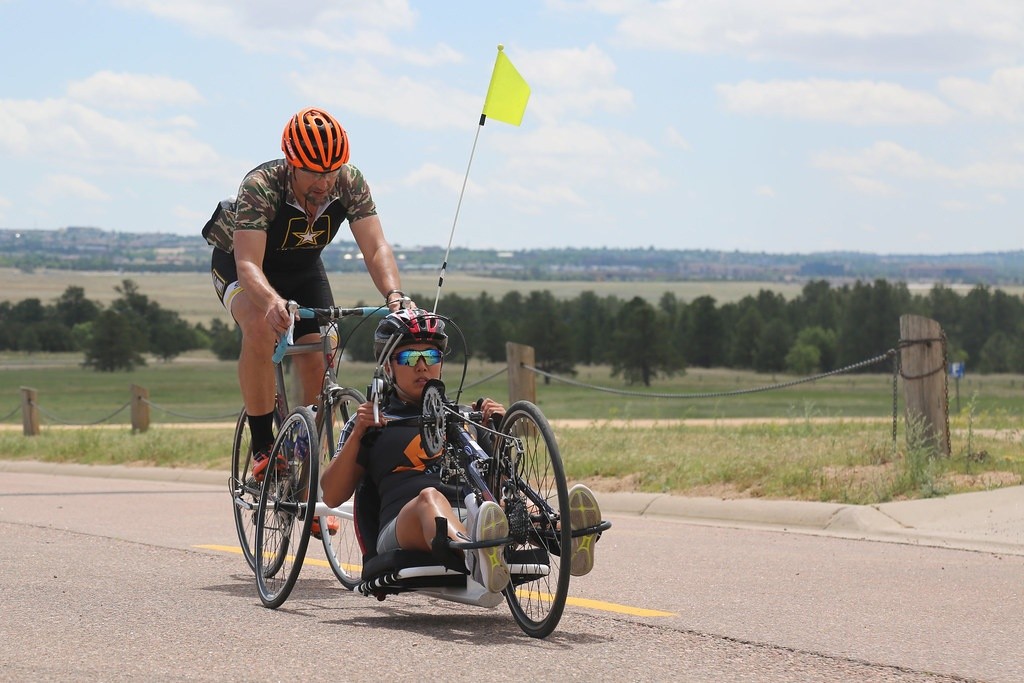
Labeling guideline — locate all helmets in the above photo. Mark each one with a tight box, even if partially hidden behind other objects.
[281,107,350,173]
[374,308,449,365]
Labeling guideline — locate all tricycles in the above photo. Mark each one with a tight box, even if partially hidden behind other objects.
[256,367,613,638]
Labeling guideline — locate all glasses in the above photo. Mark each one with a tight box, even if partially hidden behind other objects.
[306,170,340,178]
[390,349,441,367]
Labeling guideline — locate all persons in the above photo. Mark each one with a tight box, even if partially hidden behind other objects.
[202,106,405,535]
[319,309,601,593]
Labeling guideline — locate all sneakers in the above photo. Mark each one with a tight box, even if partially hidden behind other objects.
[456,501,510,593]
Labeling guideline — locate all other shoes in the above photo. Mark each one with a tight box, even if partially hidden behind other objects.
[568,484,601,577]
[311,516,338,536]
[250,445,288,482]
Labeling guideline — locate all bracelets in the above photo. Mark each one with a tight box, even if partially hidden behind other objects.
[385,290,405,302]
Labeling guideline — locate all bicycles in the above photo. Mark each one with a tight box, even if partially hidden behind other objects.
[230,297,392,608]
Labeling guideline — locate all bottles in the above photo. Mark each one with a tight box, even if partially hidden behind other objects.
[282,416,300,462]
[460,487,479,535]
[294,405,319,461]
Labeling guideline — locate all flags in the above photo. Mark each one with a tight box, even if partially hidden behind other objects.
[482,50,531,128]
